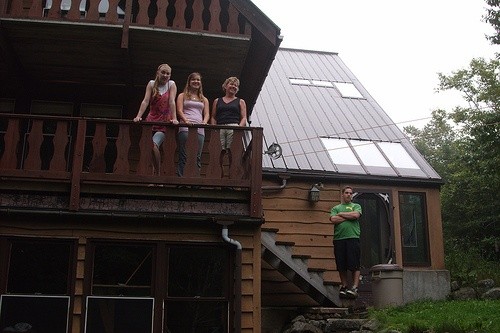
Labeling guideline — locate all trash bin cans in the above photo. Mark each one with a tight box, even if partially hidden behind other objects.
[373,265,404,308]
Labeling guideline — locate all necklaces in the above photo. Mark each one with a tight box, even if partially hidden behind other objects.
[193,95,197,99]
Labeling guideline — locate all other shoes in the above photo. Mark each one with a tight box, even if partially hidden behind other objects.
[339,283,359,297]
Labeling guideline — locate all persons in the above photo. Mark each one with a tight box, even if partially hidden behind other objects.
[330,185,362,297]
[211,77,246,178]
[133,64,179,176]
[177,73,209,176]
